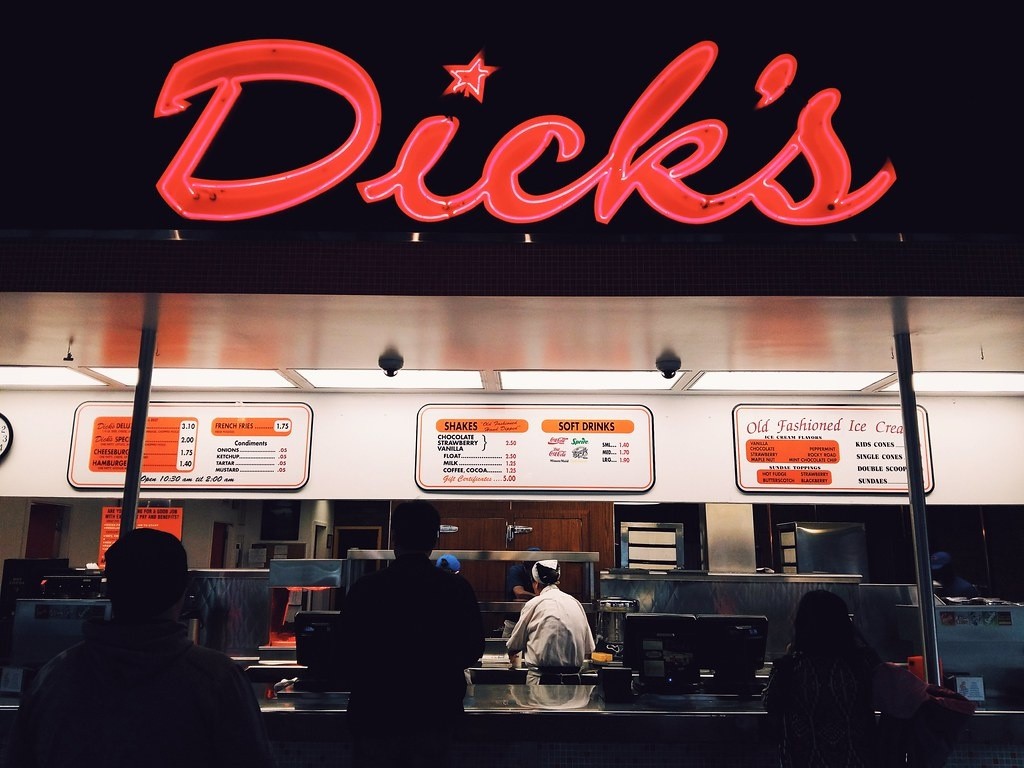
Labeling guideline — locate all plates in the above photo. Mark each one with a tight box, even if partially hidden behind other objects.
[63,567,104,576]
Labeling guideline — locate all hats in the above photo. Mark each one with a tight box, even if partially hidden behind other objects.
[930,552,952,571]
[436,554,461,572]
[524,548,541,572]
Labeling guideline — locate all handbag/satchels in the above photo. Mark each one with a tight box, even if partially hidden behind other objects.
[871,662,976,768]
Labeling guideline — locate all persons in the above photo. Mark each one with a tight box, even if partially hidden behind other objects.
[333,500,486,768]
[931,550,977,599]
[505,547,542,600]
[759,588,881,768]
[506,560,596,685]
[5,529,285,768]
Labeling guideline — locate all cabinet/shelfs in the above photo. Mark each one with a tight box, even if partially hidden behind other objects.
[345,546,604,650]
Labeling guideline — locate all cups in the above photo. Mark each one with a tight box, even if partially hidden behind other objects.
[512,651,522,669]
